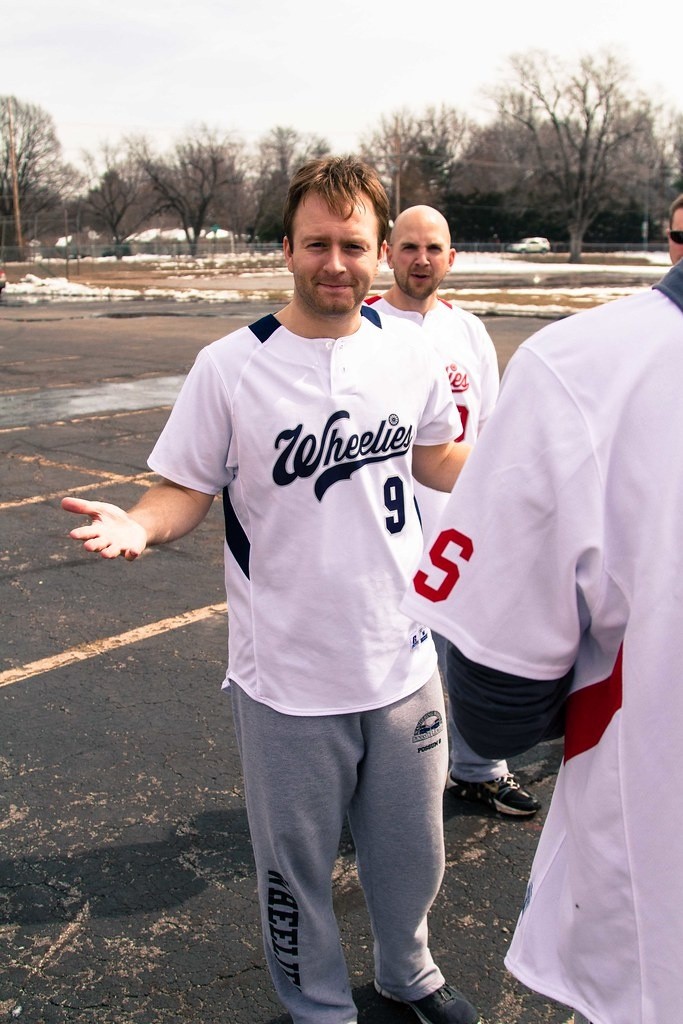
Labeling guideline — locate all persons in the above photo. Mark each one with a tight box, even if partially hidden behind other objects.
[364,205,541,816]
[666,195,683,265]
[61,161,473,1024]
[396,253,683,1024]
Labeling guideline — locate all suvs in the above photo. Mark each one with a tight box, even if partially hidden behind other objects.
[507,237,550,254]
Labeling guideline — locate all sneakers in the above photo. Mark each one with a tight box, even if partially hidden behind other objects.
[449,770,542,815]
[373,978,481,1024]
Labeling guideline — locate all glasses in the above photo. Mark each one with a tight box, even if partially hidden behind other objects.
[670,230,683,244]
